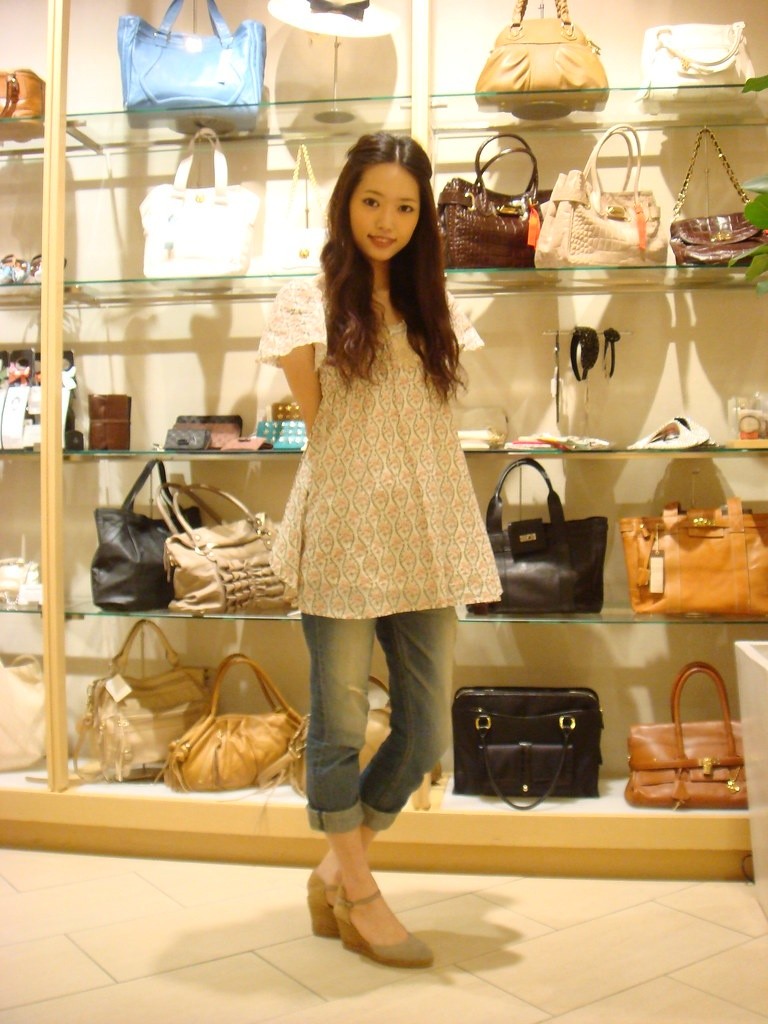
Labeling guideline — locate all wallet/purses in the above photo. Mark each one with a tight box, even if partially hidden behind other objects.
[256,419,310,451]
[222,436,266,454]
[272,404,305,423]
[86,393,133,450]
[172,414,242,449]
[163,427,212,449]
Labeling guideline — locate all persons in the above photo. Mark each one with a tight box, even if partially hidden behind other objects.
[258,130,504,967]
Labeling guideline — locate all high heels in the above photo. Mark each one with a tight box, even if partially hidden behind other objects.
[304,870,344,940]
[334,881,436,968]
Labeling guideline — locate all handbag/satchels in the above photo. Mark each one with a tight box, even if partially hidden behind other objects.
[450,683,607,810]
[435,130,554,269]
[462,457,609,616]
[158,651,303,794]
[255,672,446,811]
[639,20,762,107]
[0,654,47,776]
[474,0,610,116]
[92,456,200,612]
[68,618,219,782]
[131,127,261,283]
[625,661,751,813]
[1,381,72,451]
[155,481,301,618]
[617,494,768,623]
[257,144,334,267]
[662,125,768,267]
[529,123,675,286]
[113,0,266,130]
[0,68,47,123]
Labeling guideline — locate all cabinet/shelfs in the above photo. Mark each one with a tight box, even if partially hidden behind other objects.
[1,82,768,882]
